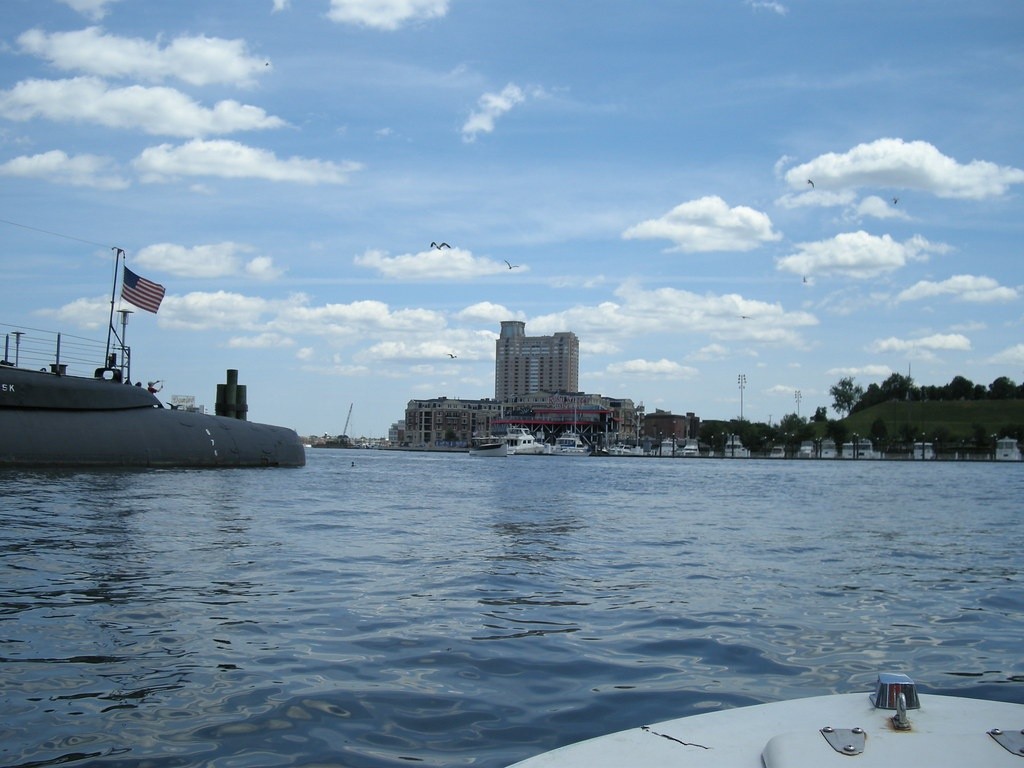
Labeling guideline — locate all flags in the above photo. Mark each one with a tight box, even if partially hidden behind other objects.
[120,265,166,314]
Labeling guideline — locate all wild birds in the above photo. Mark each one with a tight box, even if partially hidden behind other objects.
[891,197,899,204]
[504,259,519,270]
[807,179,814,188]
[431,241,451,250]
[447,353,458,358]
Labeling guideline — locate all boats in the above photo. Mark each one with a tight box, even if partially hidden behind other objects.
[0,223,310,472]
[469,398,594,457]
[608,442,657,457]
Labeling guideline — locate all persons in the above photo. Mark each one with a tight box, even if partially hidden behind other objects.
[148,380,163,395]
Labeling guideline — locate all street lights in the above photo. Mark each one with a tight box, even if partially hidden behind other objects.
[737,373,747,421]
[731,432,734,457]
[660,431,663,456]
[993,433,997,460]
[791,433,795,459]
[922,431,926,459]
[785,432,787,459]
[962,439,965,458]
[764,436,767,458]
[816,438,818,458]
[991,434,993,462]
[856,433,859,460]
[934,437,938,460]
[772,438,775,447]
[819,436,822,458]
[721,432,724,457]
[853,432,856,459]
[795,390,802,416]
[672,432,675,458]
